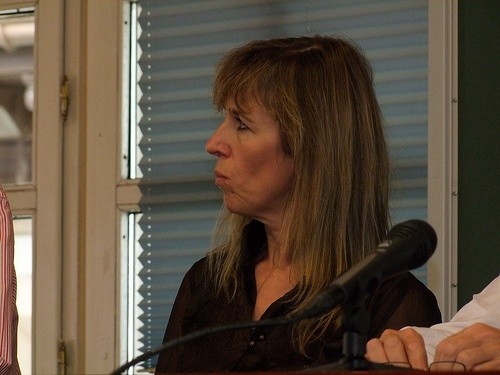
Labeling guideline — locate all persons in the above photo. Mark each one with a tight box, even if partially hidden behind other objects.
[363,274,500,373]
[152,33,442,375]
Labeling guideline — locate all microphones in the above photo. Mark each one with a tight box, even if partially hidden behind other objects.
[303,219,437,319]
